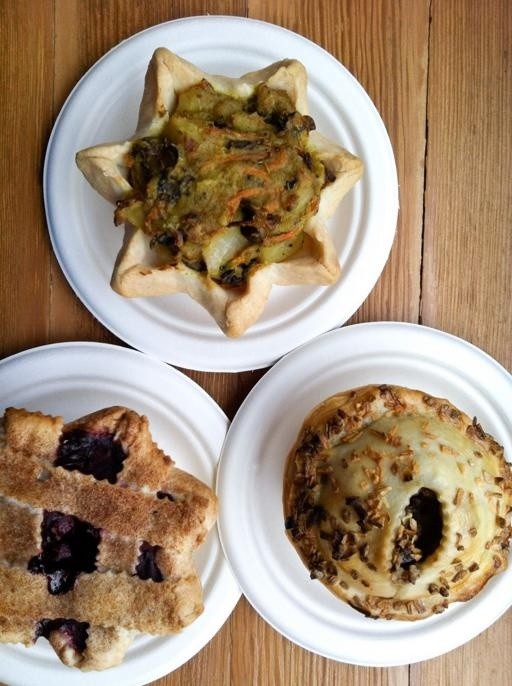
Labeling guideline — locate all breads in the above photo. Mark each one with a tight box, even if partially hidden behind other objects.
[0,405,219,673]
[279,382,511,623]
[75,46,366,342]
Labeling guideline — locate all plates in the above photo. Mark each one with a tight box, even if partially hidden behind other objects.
[1,340,242,686]
[40,12,402,378]
[213,321,512,670]
